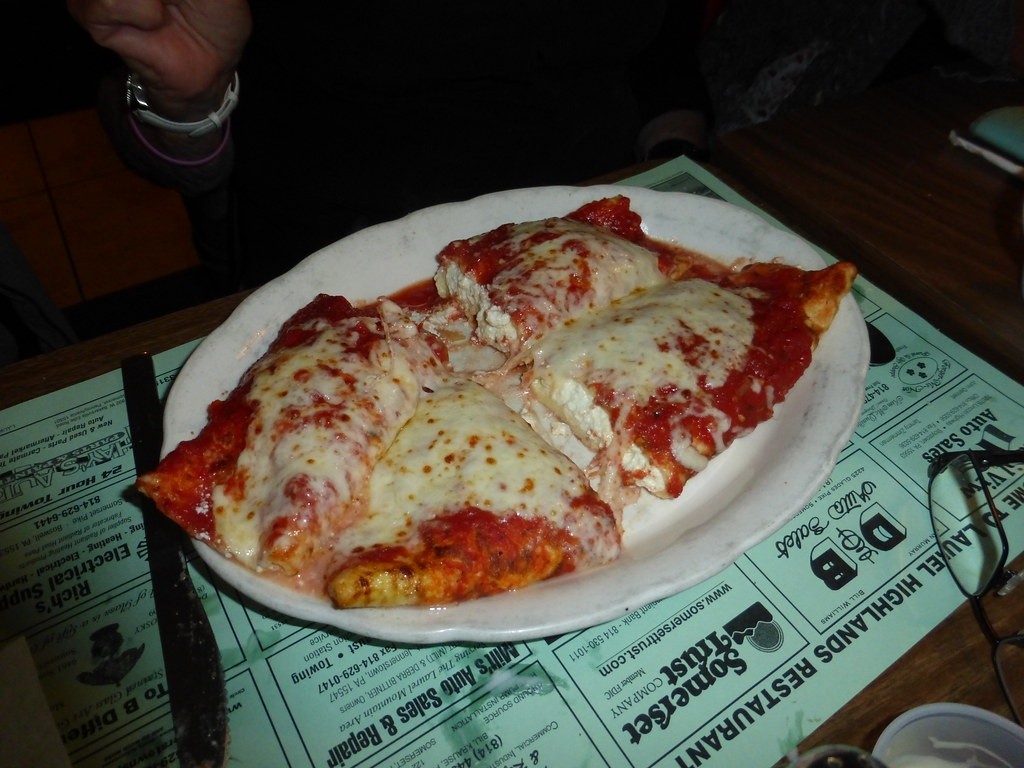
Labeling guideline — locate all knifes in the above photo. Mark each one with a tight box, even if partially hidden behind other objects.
[120,351,229,768]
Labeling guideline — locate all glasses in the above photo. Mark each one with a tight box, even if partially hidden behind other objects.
[928,447,1024,729]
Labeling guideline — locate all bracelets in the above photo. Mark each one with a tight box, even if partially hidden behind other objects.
[128,111,231,168]
[641,139,711,163]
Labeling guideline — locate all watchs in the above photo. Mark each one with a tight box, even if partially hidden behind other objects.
[122,70,240,137]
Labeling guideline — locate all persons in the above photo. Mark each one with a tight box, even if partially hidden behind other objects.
[64,0,715,272]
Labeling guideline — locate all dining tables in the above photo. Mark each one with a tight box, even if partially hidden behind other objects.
[2,0,1024,768]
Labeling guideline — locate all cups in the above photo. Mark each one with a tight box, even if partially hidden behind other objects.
[871,702,1024,768]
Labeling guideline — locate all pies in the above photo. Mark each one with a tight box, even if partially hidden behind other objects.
[433,192,858,497]
[129,292,625,610]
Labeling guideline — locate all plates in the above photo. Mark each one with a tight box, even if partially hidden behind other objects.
[159,186,871,644]
